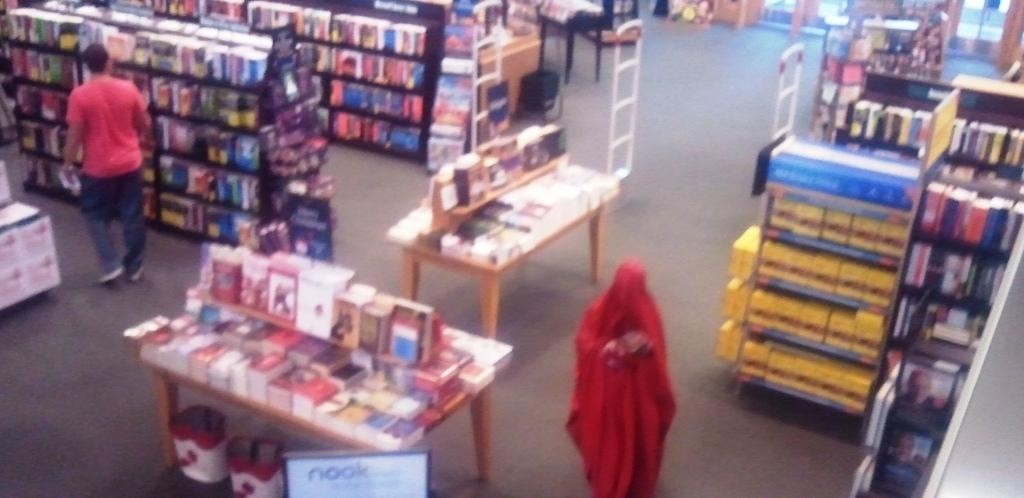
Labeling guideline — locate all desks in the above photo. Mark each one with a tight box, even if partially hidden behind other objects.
[1,203,62,318]
[381,155,623,338]
[122,288,513,485]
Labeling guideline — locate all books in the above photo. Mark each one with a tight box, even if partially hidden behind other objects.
[820,0,948,117]
[720,93,1024,498]
[412,124,620,266]
[149,2,477,173]
[133,240,513,449]
[1,1,333,250]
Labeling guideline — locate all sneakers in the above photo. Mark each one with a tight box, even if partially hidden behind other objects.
[125,262,146,283]
[96,258,123,284]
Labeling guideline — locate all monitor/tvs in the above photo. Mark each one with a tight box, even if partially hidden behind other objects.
[279,450,431,498]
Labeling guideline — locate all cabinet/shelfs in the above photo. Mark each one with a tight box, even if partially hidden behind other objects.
[9,8,152,220]
[109,0,242,21]
[715,92,959,426]
[838,70,1024,187]
[154,19,332,272]
[468,1,541,135]
[853,168,1020,497]
[244,3,447,165]
[807,10,951,146]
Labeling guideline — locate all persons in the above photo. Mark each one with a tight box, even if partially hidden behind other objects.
[60,42,154,284]
[568,263,676,497]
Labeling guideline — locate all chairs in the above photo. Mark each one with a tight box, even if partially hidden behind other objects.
[500,68,566,125]
[569,1,641,82]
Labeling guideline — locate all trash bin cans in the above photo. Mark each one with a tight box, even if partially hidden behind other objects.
[522,68,560,121]
